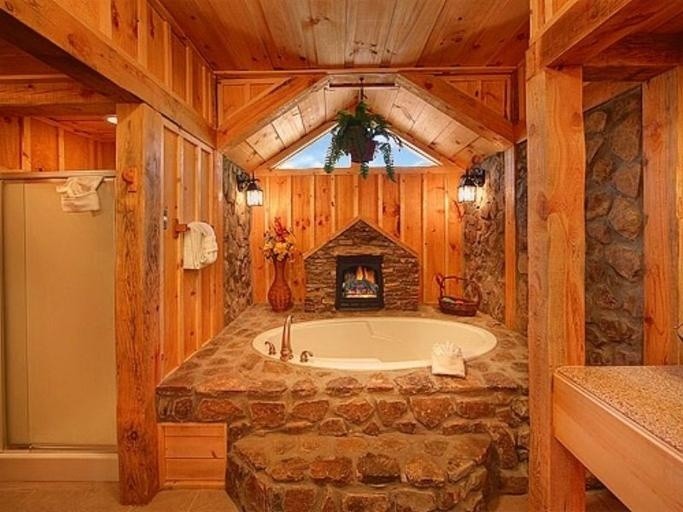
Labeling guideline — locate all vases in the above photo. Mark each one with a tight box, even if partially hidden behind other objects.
[268,257,291,312]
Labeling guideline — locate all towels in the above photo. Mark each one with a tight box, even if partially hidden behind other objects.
[431,340,466,378]
[183,221,219,270]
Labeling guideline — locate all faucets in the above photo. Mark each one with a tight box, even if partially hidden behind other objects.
[280,314,294,362]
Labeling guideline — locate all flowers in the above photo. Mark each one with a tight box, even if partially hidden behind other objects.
[262,217,297,262]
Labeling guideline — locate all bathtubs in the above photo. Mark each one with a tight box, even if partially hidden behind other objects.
[250,316,497,370]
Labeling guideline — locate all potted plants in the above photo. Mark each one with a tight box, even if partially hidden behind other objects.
[323,101,403,183]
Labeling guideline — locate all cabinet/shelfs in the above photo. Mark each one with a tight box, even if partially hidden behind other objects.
[526,0,683,512]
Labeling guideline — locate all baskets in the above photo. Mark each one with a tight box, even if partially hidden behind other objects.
[437,276,481,316]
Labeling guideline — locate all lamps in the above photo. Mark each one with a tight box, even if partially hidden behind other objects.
[457,168,485,203]
[237,172,263,206]
